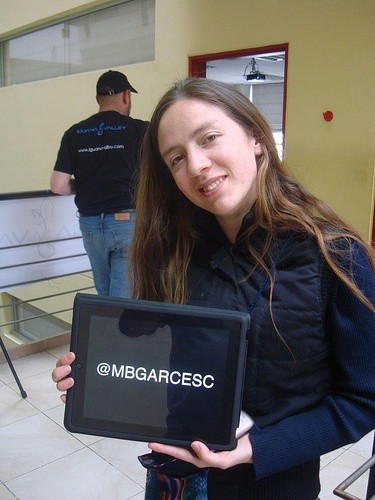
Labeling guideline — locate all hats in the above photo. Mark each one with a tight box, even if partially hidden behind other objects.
[96,70,138,96]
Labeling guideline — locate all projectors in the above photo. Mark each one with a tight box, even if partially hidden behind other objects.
[247,74,265,81]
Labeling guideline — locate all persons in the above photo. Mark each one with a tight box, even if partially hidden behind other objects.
[118,308,232,434]
[52,75,375,500]
[48,70,150,300]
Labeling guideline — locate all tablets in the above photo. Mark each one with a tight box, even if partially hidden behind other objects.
[63,292,251,451]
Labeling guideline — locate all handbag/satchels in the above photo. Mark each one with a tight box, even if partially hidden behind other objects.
[137,445,209,500]
